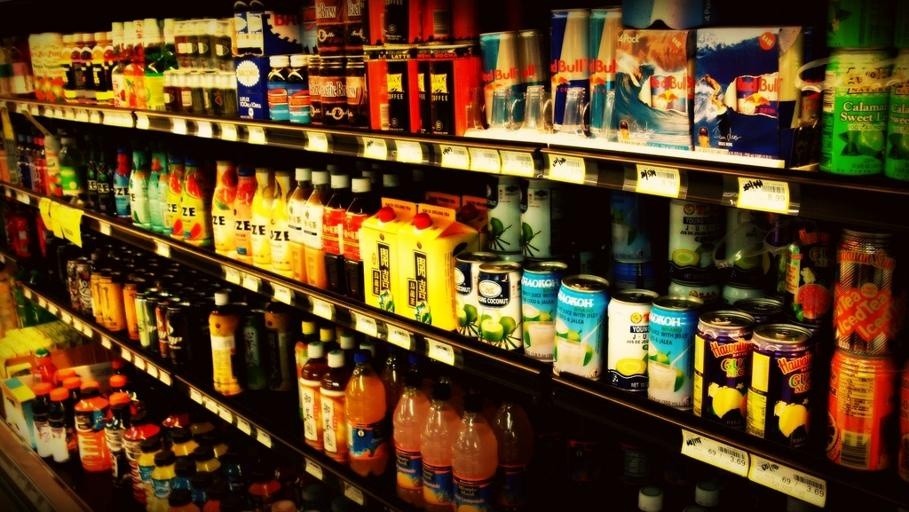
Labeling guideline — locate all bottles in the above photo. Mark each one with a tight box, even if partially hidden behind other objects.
[0,0,909,180]
[493,388,820,512]
[2,133,491,511]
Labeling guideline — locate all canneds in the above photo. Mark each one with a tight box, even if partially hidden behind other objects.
[820,47,909,181]
[67,237,269,366]
[451,197,909,484]
[307,1,625,141]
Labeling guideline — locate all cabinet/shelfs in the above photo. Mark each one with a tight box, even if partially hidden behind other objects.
[0,95,909,512]
